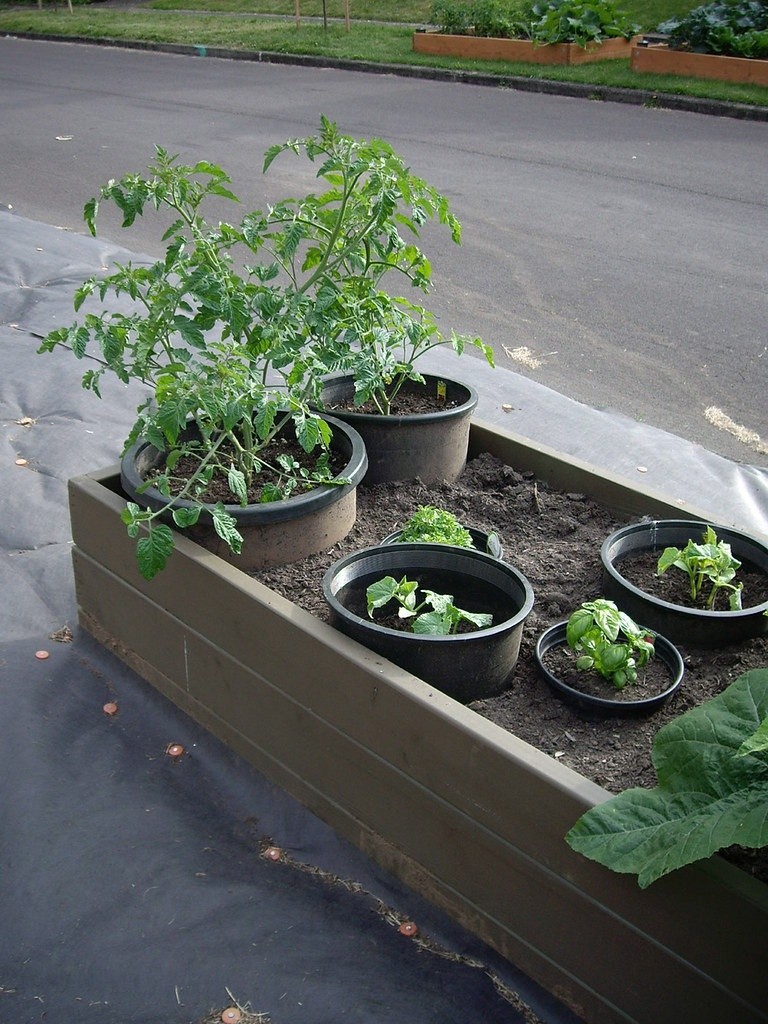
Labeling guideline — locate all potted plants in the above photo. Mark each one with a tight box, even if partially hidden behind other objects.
[39,116,462,574]
[536,597,686,719]
[379,504,501,562]
[322,543,535,702]
[305,294,494,486]
[601,518,768,653]
